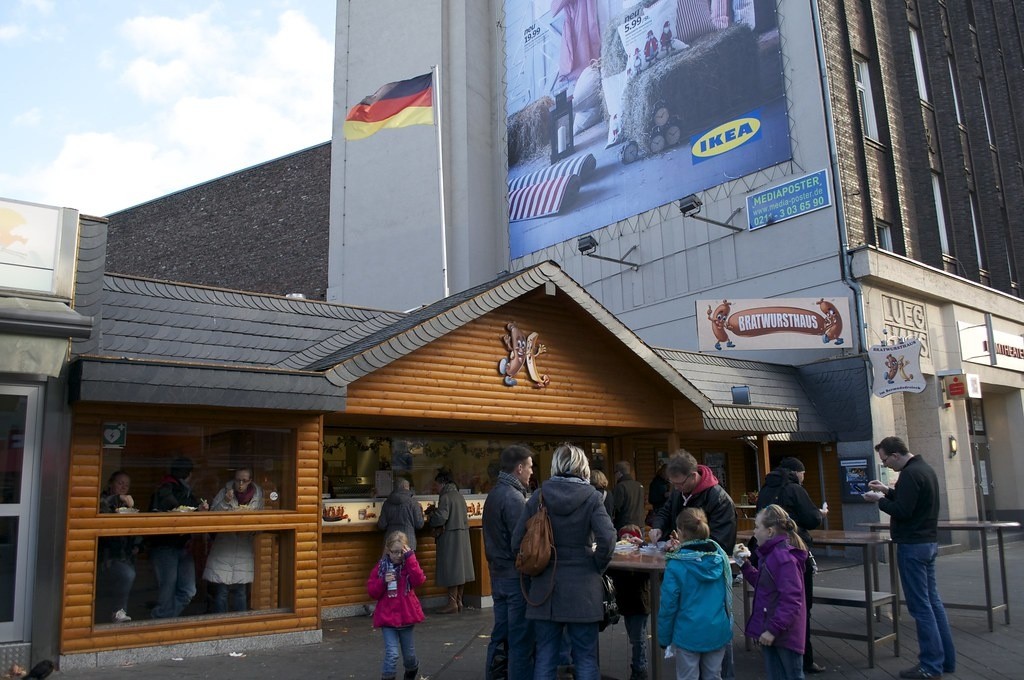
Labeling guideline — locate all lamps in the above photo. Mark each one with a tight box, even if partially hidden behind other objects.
[678,194,745,232]
[578,235,642,271]
[949,436,957,458]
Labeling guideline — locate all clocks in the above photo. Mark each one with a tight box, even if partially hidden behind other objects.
[619,140,638,164]
[650,135,666,153]
[654,107,669,126]
[666,126,680,145]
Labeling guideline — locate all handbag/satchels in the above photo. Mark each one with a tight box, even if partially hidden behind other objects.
[599,575,620,632]
[516,488,558,604]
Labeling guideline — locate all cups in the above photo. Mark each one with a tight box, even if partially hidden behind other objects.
[359,508,367,519]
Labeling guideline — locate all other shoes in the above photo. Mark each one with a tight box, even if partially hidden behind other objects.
[404,666,418,679]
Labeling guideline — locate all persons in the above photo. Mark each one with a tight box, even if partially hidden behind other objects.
[511,443,617,680]
[145,457,209,619]
[757,457,828,672]
[426,471,475,613]
[482,446,534,680]
[658,508,734,680]
[367,530,426,680]
[649,463,669,510]
[647,449,737,560]
[202,468,265,613]
[734,504,807,680]
[607,524,652,680]
[864,436,955,680]
[612,461,646,530]
[99,470,148,622]
[380,477,424,552]
[591,470,613,521]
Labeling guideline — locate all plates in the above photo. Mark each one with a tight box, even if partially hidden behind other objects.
[322,516,346,522]
[172,507,197,512]
[115,509,139,514]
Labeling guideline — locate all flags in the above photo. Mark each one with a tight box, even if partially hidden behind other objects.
[344,73,434,140]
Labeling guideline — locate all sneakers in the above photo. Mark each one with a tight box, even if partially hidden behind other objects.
[901,664,939,680]
[112,609,131,622]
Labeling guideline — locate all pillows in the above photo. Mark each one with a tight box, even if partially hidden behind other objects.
[600,0,756,148]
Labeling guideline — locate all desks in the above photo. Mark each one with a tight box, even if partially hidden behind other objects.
[596,542,744,680]
[737,529,900,668]
[857,521,1020,634]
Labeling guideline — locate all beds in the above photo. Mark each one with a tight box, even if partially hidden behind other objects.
[599,22,760,156]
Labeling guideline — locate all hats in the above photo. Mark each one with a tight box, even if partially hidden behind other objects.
[780,457,805,472]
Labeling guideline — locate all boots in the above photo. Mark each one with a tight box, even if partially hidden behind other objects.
[435,586,458,614]
[458,586,463,611]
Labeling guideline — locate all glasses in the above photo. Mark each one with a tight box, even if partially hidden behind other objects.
[391,549,403,555]
[236,478,250,483]
[669,474,691,485]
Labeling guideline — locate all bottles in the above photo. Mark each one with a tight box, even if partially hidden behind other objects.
[387,563,398,597]
